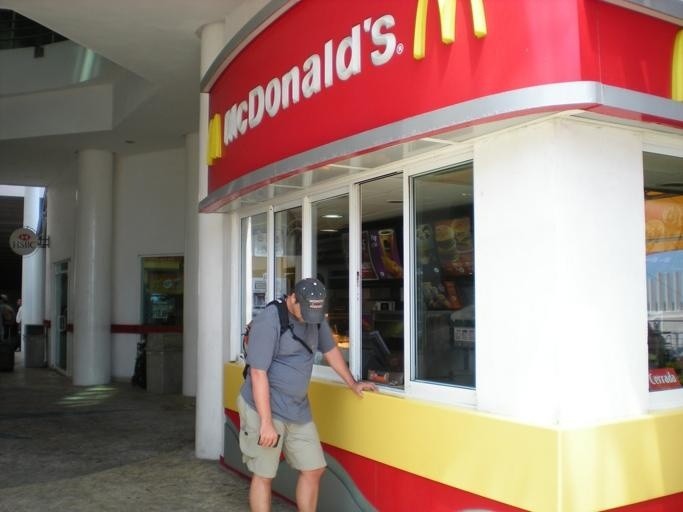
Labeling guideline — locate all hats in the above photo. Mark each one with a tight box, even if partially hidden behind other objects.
[295,279,327,324]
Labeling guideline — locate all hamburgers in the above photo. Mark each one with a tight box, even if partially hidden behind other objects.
[450,217,474,253]
[433,224,460,263]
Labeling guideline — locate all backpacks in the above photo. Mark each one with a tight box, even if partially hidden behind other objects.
[242,299,289,379]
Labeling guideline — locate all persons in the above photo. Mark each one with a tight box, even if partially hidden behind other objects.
[235,278,380,511]
[0,293,16,372]
[16,296,23,350]
